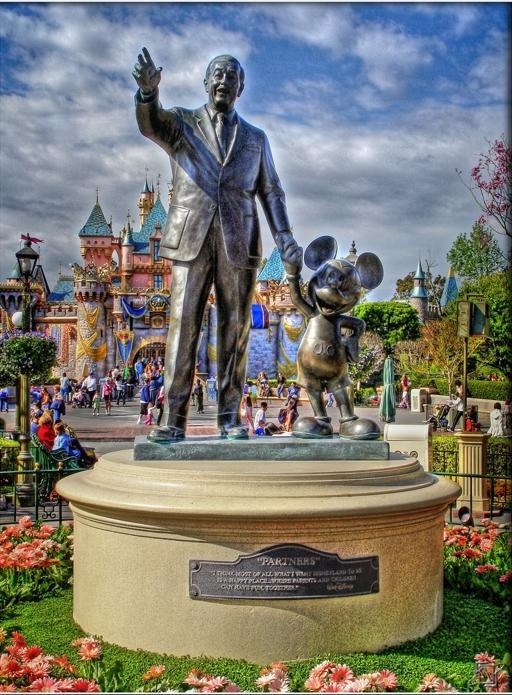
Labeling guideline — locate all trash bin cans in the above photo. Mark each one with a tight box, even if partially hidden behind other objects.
[206,379,218,402]
[411,389,428,412]
[114,383,134,399]
[467,405,478,423]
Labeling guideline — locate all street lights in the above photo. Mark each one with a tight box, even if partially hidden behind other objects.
[11,230,41,507]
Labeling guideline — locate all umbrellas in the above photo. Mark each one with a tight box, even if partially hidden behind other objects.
[379,354,395,422]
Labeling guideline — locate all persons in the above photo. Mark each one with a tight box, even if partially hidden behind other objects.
[400,375,413,413]
[448,381,466,433]
[488,403,508,438]
[132,46,297,441]
